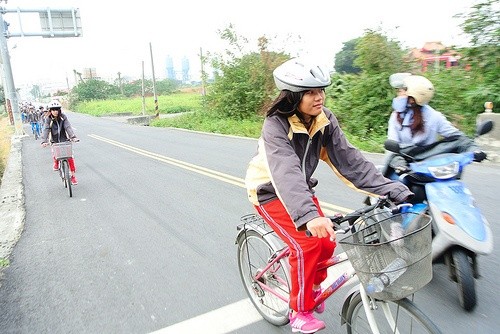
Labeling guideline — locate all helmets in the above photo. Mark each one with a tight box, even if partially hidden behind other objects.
[272,57,331,93]
[22,103,43,110]
[48,100,61,108]
[403,75,434,105]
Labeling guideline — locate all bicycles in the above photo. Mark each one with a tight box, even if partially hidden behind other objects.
[234,192,441,334]
[21,111,45,141]
[42,138,80,197]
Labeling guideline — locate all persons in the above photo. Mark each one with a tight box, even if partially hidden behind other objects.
[245,58,417,334]
[41,101,79,186]
[18,100,50,141]
[378,71,488,227]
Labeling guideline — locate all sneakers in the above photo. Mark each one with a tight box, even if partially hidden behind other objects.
[289,308,326,334]
[312,286,324,313]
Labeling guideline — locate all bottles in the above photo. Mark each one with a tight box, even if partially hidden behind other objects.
[367,258,408,292]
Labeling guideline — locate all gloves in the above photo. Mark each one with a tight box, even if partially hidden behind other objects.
[469,149,486,163]
[390,156,411,175]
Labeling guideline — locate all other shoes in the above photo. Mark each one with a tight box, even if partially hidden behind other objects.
[70,176,77,185]
[53,162,59,171]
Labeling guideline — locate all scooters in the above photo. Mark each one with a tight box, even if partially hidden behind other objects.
[365,119,493,312]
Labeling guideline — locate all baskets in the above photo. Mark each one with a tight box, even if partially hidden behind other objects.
[338,211,433,301]
[51,141,72,160]
